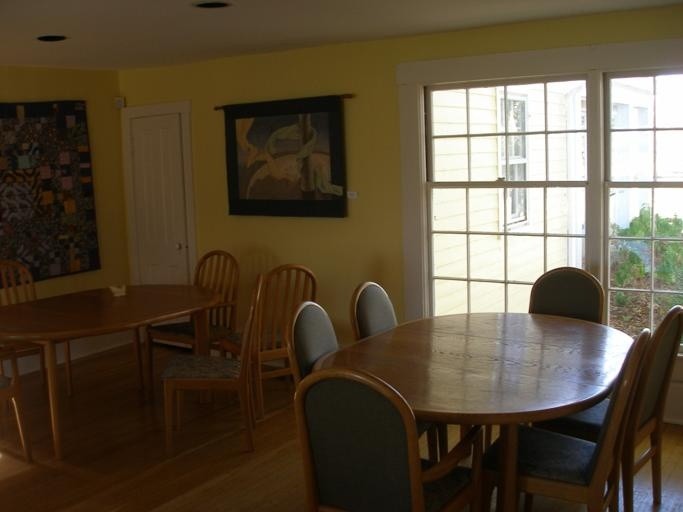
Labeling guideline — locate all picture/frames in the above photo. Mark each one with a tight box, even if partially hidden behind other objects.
[213,93,355,218]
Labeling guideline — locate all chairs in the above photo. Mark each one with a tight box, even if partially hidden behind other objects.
[478,329,648,512]
[144,249,239,406]
[293,370,485,510]
[349,280,468,460]
[0,372,32,462]
[529,267,602,324]
[159,273,265,453]
[291,301,436,463]
[564,303,681,512]
[0,263,73,396]
[221,265,318,418]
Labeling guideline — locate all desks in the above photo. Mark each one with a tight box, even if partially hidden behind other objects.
[1,285,220,451]
[309,312,632,510]
